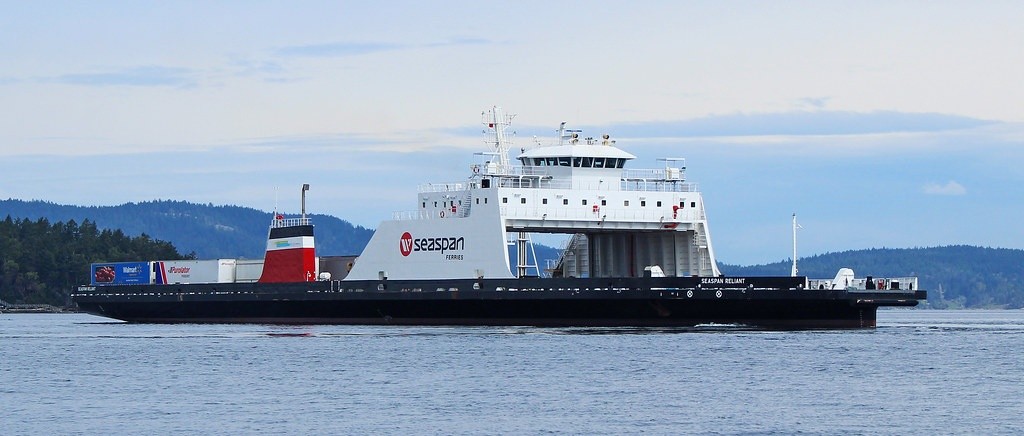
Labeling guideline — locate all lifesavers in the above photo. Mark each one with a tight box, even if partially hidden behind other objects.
[440,211,444,218]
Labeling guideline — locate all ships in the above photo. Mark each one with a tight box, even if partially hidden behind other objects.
[68,102,930,327]
